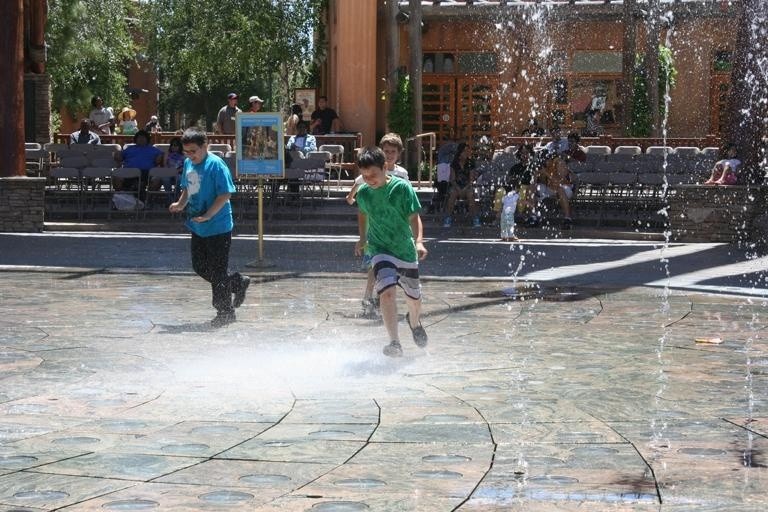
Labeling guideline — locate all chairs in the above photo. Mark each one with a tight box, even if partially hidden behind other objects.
[474,141,719,209]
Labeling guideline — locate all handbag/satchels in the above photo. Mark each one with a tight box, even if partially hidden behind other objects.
[112,194,144,211]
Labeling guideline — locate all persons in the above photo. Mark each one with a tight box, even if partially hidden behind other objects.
[69,95,344,211]
[354,145,429,356]
[705,143,741,185]
[346,132,408,307]
[436,110,607,241]
[169,126,249,328]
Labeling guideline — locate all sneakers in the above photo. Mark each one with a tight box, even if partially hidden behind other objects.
[523,218,538,227]
[443,216,452,227]
[406,313,427,348]
[384,342,403,358]
[234,277,250,308]
[473,218,481,230]
[558,219,575,231]
[212,314,237,326]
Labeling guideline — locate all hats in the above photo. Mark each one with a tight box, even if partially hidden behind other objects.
[249,96,264,103]
[228,93,238,99]
[118,107,136,120]
